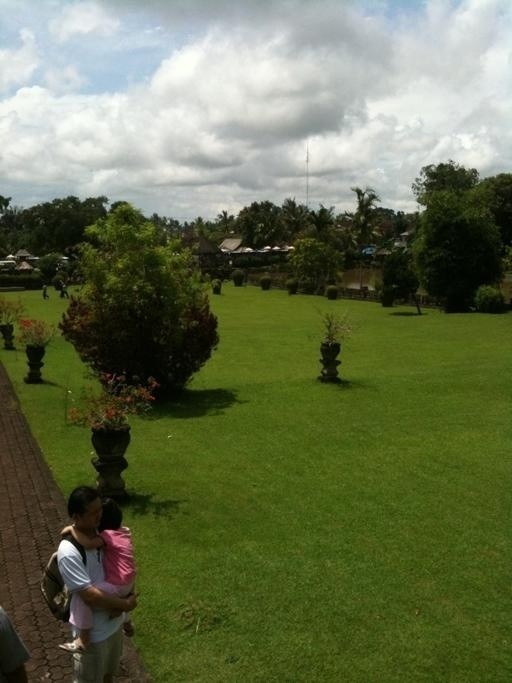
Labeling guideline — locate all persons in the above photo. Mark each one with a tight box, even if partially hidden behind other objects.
[57,485,139,683]
[43,286,49,300]
[59,280,69,299]
[0,606,32,683]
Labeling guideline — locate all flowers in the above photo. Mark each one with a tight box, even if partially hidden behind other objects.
[1,296,59,350]
[58,359,165,431]
[307,302,361,344]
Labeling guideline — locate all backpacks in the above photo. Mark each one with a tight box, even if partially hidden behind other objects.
[40,535,87,621]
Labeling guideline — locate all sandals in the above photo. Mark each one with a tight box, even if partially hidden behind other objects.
[121,620,135,637]
[58,641,89,655]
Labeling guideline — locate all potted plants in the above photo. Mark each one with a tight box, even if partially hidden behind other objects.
[209,269,338,301]
